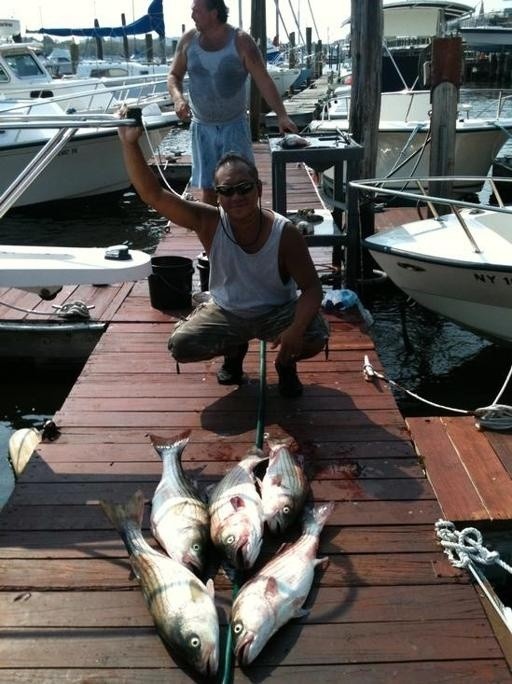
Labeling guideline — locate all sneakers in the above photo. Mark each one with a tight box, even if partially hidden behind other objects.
[275,360,303,397]
[217,341,248,384]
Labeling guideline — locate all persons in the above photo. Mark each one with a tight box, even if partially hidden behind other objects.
[114,102,334,397]
[166,0,301,212]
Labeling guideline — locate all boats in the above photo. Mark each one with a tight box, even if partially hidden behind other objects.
[1,17,187,207]
[456,23,510,51]
[0,109,152,285]
[302,0,511,194]
[62,59,300,96]
[343,171,512,346]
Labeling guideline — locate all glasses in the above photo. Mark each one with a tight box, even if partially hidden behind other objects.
[215,179,257,195]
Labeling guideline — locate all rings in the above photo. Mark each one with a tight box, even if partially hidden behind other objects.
[291,354,295,358]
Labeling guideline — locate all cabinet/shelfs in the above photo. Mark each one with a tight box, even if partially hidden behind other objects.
[267,131,365,292]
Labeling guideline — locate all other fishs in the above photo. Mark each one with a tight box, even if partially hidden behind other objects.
[203,445,270,571]
[150,429,210,580]
[99,489,220,682]
[231,500,336,665]
[255,436,309,536]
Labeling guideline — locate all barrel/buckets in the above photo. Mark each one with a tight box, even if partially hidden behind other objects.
[197,251,210,292]
[148,256,196,309]
